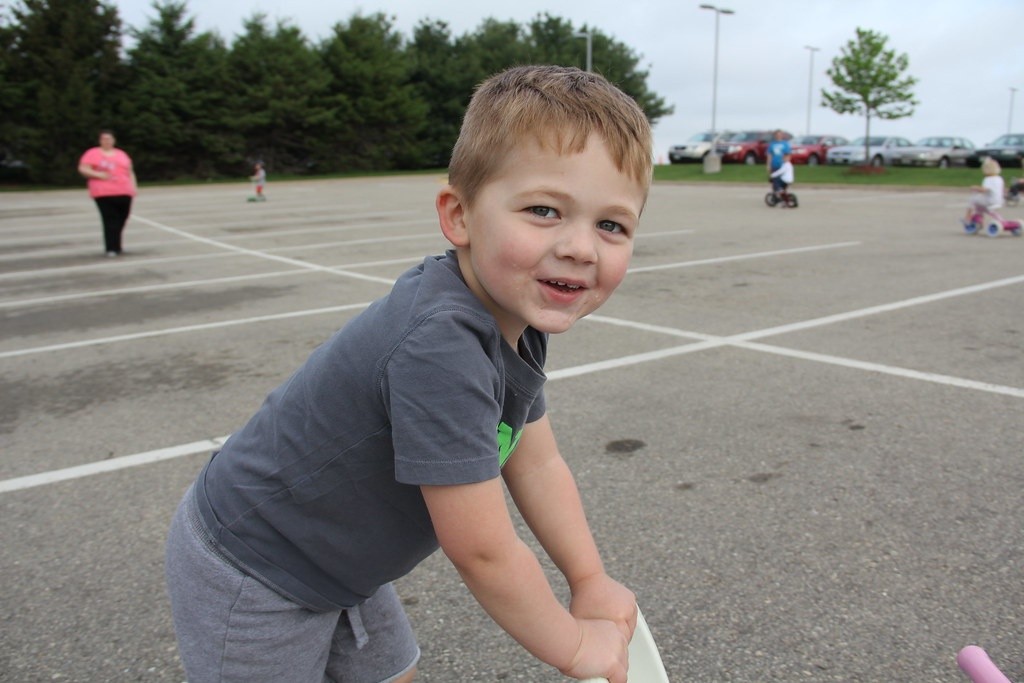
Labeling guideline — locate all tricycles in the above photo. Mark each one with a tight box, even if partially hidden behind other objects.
[961,187,1024,238]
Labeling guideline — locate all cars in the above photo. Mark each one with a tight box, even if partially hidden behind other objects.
[786,134,850,166]
[894,135,978,170]
[826,134,913,170]
[668,130,723,164]
[720,129,794,165]
[975,133,1024,170]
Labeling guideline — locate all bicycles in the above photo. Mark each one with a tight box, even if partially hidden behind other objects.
[765,178,798,209]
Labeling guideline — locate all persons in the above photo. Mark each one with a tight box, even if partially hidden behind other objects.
[164,65,654,682]
[1009,154,1024,202]
[765,130,796,204]
[249,159,267,199]
[968,157,1006,218]
[78,130,137,256]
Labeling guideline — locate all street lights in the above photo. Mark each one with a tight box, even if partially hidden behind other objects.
[571,32,602,74]
[804,44,821,134]
[1006,86,1018,133]
[701,4,735,174]
